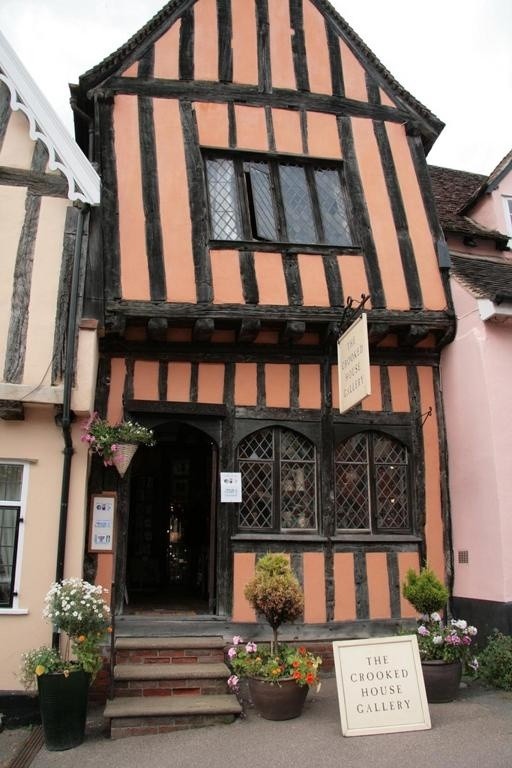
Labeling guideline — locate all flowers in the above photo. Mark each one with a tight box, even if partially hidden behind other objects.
[414,610,480,667]
[223,635,322,695]
[15,575,111,689]
[81,414,155,472]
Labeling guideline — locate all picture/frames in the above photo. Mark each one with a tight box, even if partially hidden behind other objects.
[86,492,119,555]
[330,633,434,737]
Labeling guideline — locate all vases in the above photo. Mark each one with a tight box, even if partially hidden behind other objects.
[422,657,465,704]
[35,661,87,752]
[248,674,313,720]
